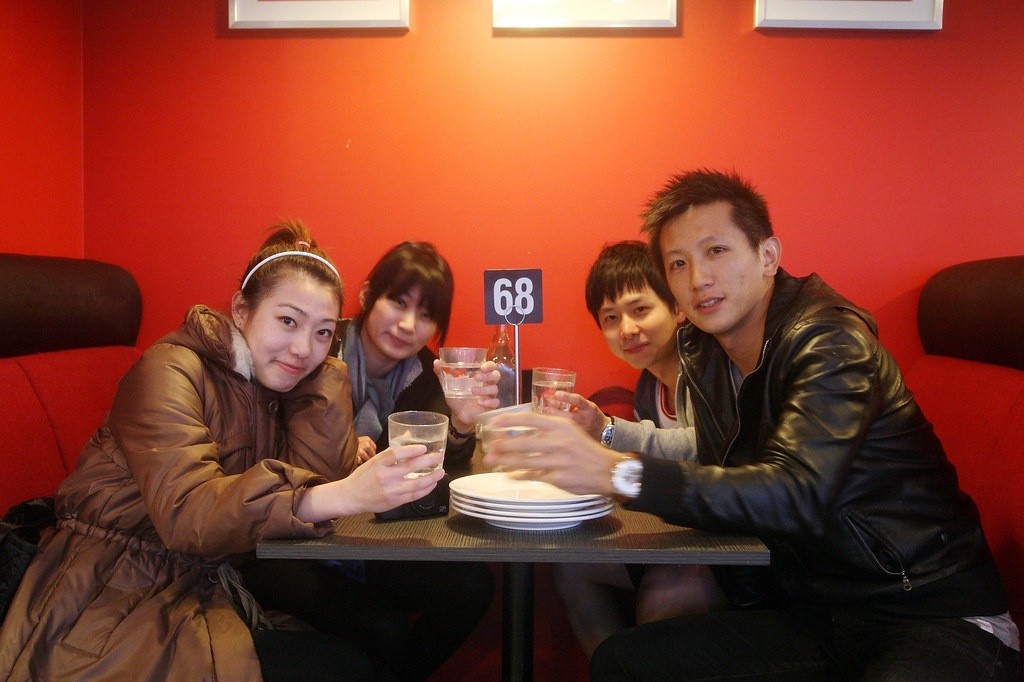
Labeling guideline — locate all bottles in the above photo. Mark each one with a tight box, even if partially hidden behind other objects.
[488,324,517,407]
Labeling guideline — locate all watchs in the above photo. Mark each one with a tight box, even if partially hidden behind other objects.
[600,416,615,448]
[611,455,643,499]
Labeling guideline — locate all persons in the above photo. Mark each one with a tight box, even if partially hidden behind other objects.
[481,168,1024,682]
[0,219,446,682]
[241,242,497,682]
[542,239,714,660]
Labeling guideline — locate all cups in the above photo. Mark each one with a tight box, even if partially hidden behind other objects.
[388,411,449,481]
[531,368,576,415]
[439,346,488,399]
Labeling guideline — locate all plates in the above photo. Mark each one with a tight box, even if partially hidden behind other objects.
[449,473,614,531]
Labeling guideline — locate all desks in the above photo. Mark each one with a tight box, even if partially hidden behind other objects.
[257,440,770,682]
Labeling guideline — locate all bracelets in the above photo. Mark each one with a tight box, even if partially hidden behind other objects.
[447,415,477,440]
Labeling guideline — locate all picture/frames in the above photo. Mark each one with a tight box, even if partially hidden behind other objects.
[229,0,409,29]
[755,0,943,29]
[492,0,677,27]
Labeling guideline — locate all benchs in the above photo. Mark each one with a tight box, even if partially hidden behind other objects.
[0,252,142,618]
[903,256,1024,641]
